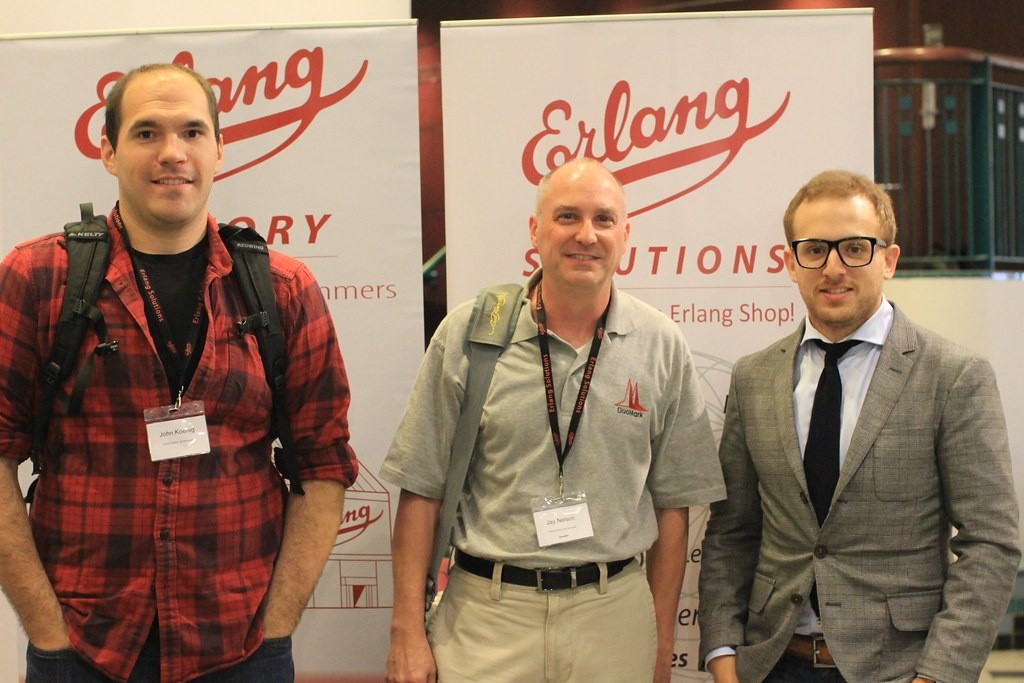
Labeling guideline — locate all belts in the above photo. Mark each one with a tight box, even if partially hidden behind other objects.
[784,633,841,668]
[454,548,635,591]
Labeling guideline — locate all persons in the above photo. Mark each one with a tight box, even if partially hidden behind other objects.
[377,157,727,683]
[694,168,1022,683]
[0,66,361,683]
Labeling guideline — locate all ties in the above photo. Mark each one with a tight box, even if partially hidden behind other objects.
[802,340,865,528]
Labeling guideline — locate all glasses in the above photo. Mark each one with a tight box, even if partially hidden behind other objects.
[790,236,888,270]
